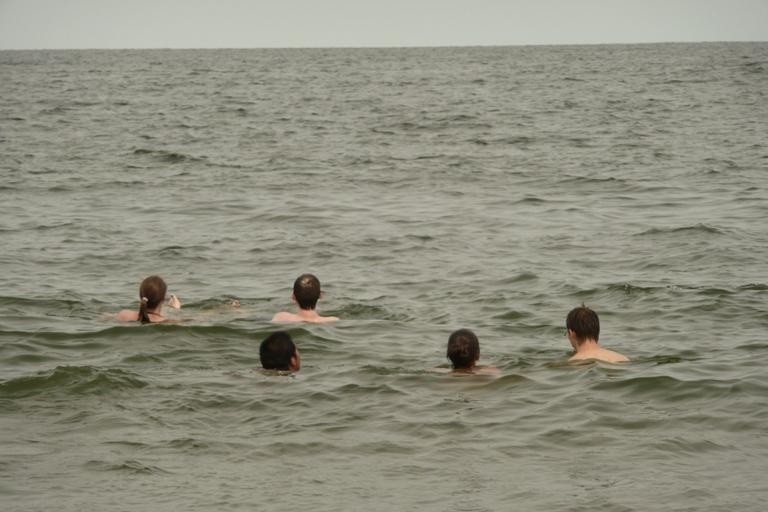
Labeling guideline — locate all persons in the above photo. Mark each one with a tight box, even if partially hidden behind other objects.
[260,330,301,374]
[102,275,181,325]
[270,273,341,325]
[432,328,499,379]
[564,299,629,364]
